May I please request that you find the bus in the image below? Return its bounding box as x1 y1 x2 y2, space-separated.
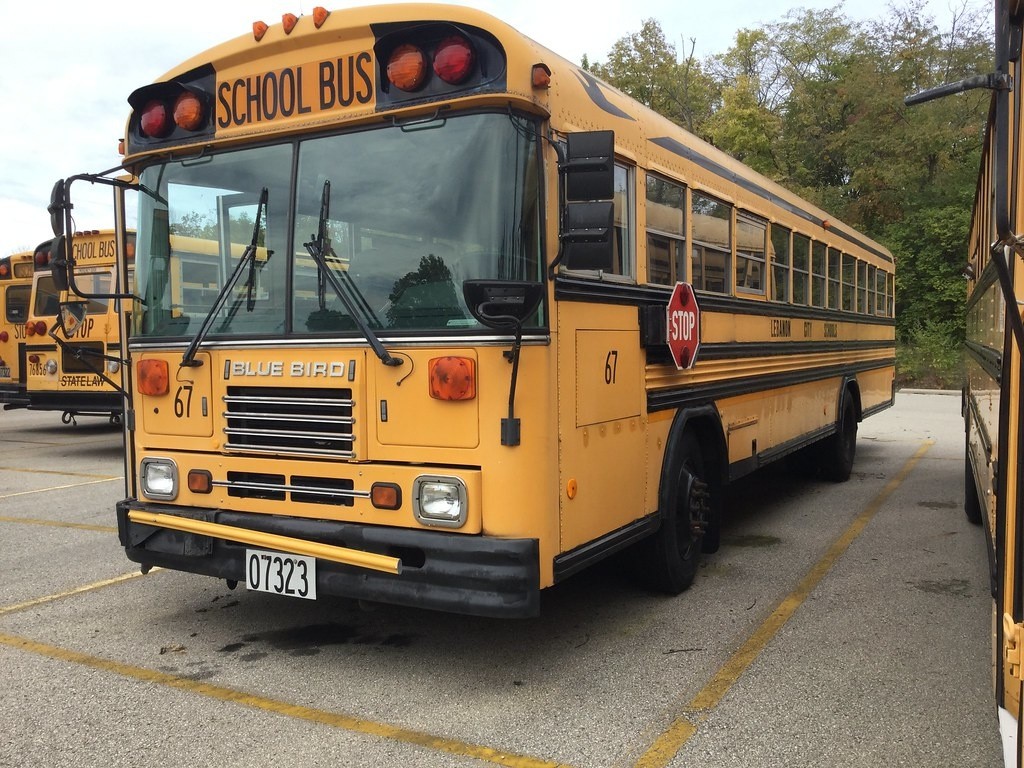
20 229 350 429
907 0 1019 768
45 4 902 626
0 247 142 412
